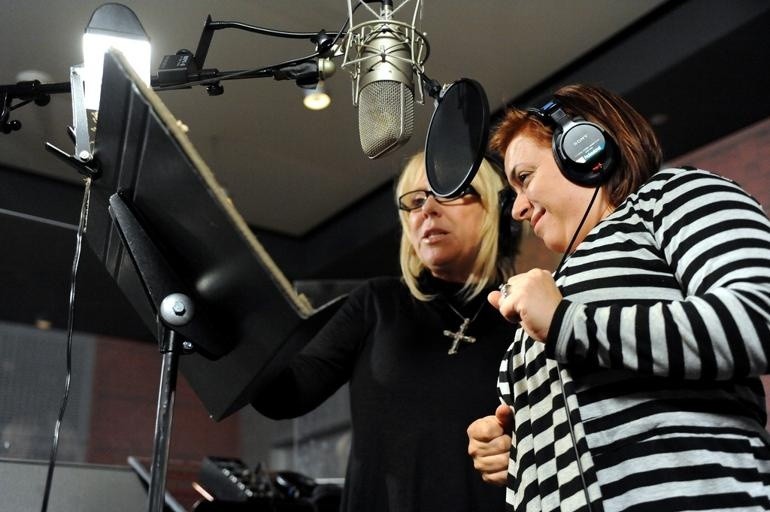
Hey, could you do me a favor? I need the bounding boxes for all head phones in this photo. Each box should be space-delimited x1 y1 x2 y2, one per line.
466 145 522 244
528 103 616 187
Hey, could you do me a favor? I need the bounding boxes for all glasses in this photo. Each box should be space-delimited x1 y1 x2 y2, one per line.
399 184 478 210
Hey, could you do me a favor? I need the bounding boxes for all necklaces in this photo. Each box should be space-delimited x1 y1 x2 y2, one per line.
438 290 489 358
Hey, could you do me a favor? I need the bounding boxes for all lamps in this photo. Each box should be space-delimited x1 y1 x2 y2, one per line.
303 80 331 112
69 3 152 163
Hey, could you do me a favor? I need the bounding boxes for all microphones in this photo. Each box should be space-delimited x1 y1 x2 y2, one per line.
356 0 416 162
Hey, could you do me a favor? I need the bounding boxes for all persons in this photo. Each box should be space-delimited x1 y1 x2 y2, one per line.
251 149 512 511
465 83 770 512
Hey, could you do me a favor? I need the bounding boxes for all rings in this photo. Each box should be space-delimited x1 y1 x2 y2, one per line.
498 281 511 299
